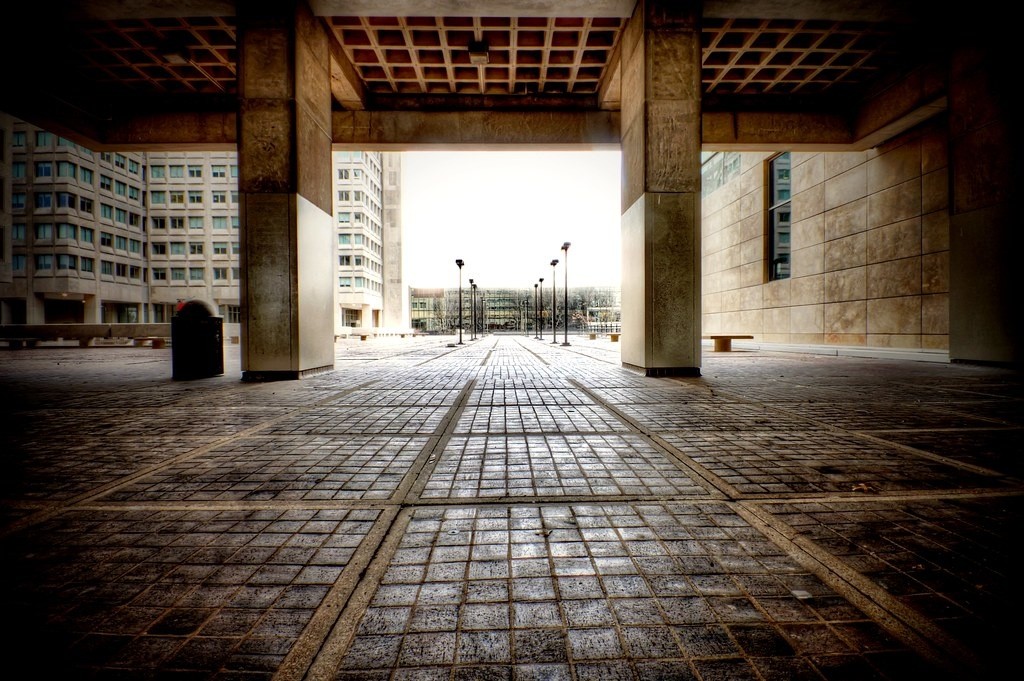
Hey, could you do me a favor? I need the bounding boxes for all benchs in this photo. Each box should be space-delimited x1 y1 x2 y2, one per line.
588 332 602 340
607 334 620 342
134 337 171 350
702 335 754 351
334 333 430 342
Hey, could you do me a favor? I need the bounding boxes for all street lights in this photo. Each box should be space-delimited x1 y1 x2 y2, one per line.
472 284 477 339
538 277 544 340
551 259 560 344
468 278 474 341
534 283 539 339
455 258 464 345
561 242 571 345
